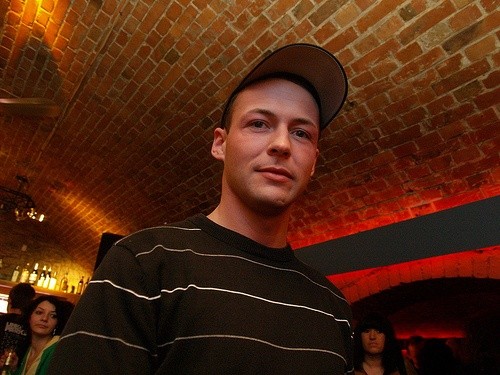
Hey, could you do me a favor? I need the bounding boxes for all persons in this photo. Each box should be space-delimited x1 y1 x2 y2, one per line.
354 316 458 375
0 283 74 375
47 43 356 375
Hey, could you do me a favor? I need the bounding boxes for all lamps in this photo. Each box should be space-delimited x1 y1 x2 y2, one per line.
0 176 45 223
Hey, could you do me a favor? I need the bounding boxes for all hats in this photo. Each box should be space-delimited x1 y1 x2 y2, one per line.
220 42 350 132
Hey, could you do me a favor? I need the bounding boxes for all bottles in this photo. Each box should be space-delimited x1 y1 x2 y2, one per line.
37 265 76 296
28 262 39 285
84 278 90 291
76 276 84 295
0 346 16 375
19 262 30 284
10 265 20 283
